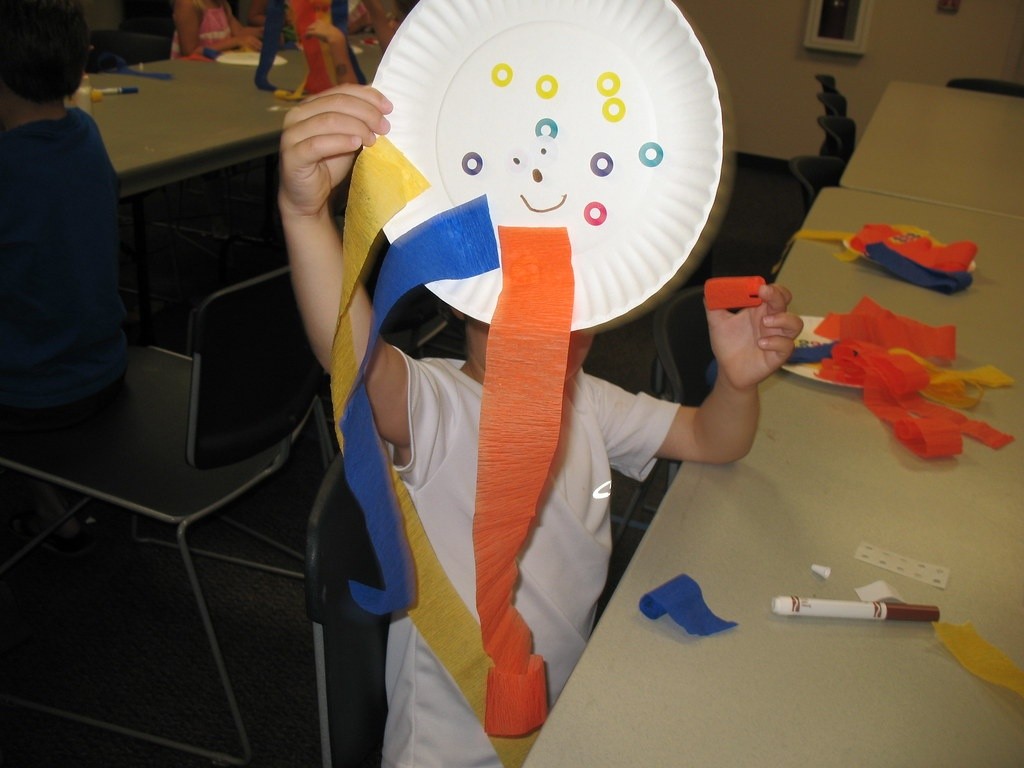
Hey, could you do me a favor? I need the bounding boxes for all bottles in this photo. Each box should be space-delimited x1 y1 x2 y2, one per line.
74 77 93 115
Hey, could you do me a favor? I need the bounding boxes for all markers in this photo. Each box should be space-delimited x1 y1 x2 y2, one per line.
94 85 138 97
773 597 941 624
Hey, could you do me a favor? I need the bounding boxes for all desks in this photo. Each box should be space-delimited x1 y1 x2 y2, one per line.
61 75 299 198
117 29 382 100
520 186 1024 767
838 80 1024 219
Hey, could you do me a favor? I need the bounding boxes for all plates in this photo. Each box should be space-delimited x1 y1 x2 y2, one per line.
843 239 976 271
782 316 862 388
364 0 724 333
217 52 287 66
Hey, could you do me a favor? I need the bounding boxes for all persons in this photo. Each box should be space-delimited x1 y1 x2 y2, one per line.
169 0 284 239
276 84 805 768
306 0 419 86
1 0 130 557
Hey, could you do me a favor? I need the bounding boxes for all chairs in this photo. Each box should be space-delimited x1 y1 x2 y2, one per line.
304 454 388 768
611 73 858 547
0 262 334 764
